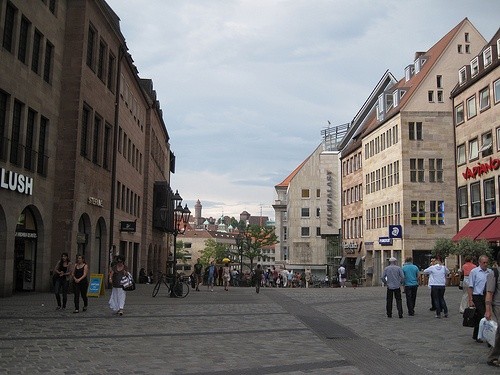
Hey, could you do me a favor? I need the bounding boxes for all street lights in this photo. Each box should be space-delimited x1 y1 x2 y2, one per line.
160 189 191 298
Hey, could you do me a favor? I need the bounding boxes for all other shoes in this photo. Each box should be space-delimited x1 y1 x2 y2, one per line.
83 304 88 311
399 315 403 318
473 337 484 343
61 307 65 312
56 305 61 310
488 360 500 367
429 307 437 311
120 311 123 315
387 314 392 318
75 310 79 313
435 314 441 318
444 313 447 317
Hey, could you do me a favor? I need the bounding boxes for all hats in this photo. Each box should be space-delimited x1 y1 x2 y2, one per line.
389 257 397 262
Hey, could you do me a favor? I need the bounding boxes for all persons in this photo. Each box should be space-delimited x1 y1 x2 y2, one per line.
222 263 230 292
337 265 347 288
484 253 499 367
139 268 150 285
468 255 493 343
253 265 264 294
54 253 72 311
422 255 449 318
401 257 420 316
380 257 405 318
72 255 89 313
460 254 477 313
261 267 313 288
205 260 217 292
192 258 204 292
108 255 129 316
232 269 240 287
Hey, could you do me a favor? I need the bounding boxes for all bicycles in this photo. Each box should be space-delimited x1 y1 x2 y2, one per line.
151 271 190 300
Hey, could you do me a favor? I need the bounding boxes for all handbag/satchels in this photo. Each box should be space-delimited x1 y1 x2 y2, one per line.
477 317 498 348
463 305 479 327
120 271 136 291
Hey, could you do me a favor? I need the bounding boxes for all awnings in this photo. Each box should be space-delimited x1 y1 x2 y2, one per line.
451 217 496 245
475 216 500 242
